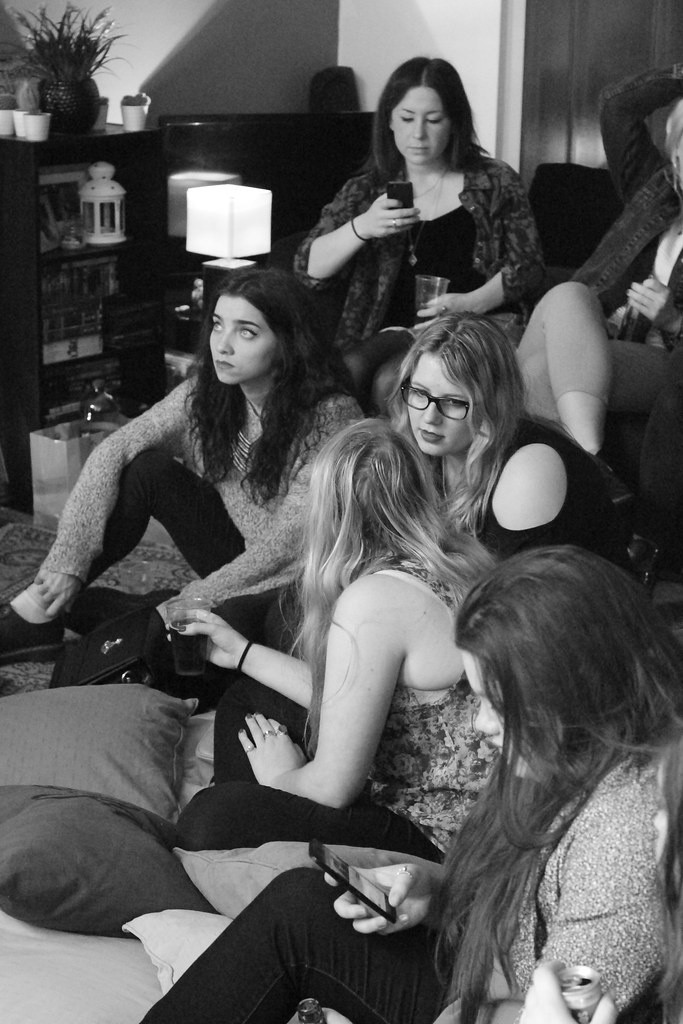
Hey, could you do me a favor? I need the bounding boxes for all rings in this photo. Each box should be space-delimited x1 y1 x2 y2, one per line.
245 745 254 753
393 219 396 227
276 725 288 736
397 866 413 878
263 731 277 740
441 306 447 313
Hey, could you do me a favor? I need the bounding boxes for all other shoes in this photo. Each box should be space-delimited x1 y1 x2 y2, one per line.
0 601 65 665
588 447 635 506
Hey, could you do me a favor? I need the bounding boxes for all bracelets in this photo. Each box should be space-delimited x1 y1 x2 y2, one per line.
351 217 368 241
236 641 256 672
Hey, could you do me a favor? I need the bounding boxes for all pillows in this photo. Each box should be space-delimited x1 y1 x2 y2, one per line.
2 686 439 1023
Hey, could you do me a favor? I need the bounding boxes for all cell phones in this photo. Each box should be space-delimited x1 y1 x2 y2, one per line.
387 181 415 211
308 837 398 926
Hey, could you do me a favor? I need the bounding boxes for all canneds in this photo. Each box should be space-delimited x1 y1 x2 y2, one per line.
554 965 602 1024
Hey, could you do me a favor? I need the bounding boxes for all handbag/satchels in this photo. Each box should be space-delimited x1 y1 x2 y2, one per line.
49 601 166 688
28 420 119 535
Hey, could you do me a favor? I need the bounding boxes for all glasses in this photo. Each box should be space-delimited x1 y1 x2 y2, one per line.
400 375 477 420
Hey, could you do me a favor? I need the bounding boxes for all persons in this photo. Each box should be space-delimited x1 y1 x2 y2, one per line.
133 546 683 1024
0 56 683 667
165 418 503 863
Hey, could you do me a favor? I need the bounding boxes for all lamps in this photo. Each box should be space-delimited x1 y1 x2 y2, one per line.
186 184 274 340
78 161 128 244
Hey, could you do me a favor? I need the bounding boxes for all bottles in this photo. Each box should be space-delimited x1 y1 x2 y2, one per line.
297 998 327 1024
81 379 123 438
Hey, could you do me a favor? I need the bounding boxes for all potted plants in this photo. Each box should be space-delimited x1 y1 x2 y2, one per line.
120 93 149 133
1 77 53 140
92 97 109 131
10 5 131 134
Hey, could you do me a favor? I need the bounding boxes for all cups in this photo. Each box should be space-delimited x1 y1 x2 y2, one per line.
165 597 212 675
412 274 452 323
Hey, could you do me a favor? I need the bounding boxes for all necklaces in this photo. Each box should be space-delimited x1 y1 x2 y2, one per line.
441 457 448 503
246 399 260 418
407 168 448 265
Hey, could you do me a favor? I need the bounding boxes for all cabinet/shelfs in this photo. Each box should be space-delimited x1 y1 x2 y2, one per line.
2 109 374 511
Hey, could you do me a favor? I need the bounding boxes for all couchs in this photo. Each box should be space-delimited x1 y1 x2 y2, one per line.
530 160 682 523
